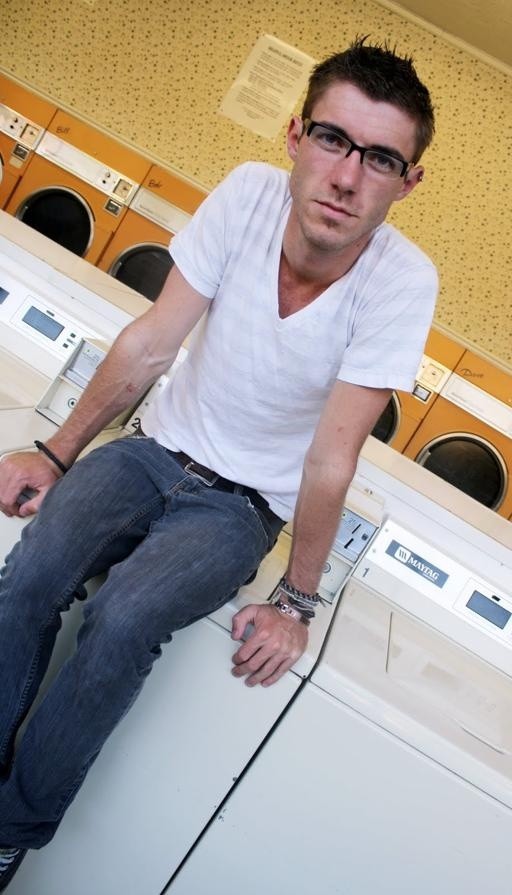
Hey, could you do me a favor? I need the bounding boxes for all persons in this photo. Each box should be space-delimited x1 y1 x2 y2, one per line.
0 34 439 895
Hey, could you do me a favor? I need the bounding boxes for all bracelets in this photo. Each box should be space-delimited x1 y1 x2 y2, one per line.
33 439 69 475
267 577 331 627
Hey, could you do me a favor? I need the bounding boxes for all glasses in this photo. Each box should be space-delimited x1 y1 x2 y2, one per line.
300 115 408 185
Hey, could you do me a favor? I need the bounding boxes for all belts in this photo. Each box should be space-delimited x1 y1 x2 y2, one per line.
134 424 287 531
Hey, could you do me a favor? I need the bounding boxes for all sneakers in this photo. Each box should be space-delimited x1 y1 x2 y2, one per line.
0 844 27 892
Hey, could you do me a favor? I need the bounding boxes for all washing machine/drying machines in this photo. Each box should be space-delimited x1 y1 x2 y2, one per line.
2 69 511 519
3 207 511 894
